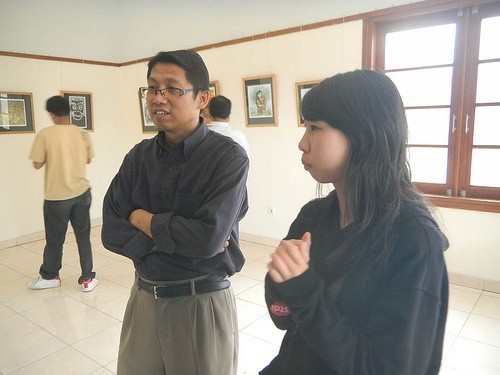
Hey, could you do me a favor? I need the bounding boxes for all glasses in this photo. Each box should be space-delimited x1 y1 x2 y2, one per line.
138 87 198 101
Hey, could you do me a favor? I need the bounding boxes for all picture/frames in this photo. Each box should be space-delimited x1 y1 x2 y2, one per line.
198 80 221 128
295 80 322 128
61 91 94 133
242 74 278 128
0 91 36 134
140 87 161 134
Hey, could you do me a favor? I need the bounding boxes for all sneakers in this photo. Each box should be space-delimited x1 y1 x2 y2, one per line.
81 278 99 291
28 275 62 289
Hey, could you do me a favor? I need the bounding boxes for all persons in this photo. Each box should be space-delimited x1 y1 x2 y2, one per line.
259 69 450 375
101 49 250 375
204 96 249 159
27 96 99 292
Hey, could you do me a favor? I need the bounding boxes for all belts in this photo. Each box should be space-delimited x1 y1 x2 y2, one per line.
135 271 230 300
